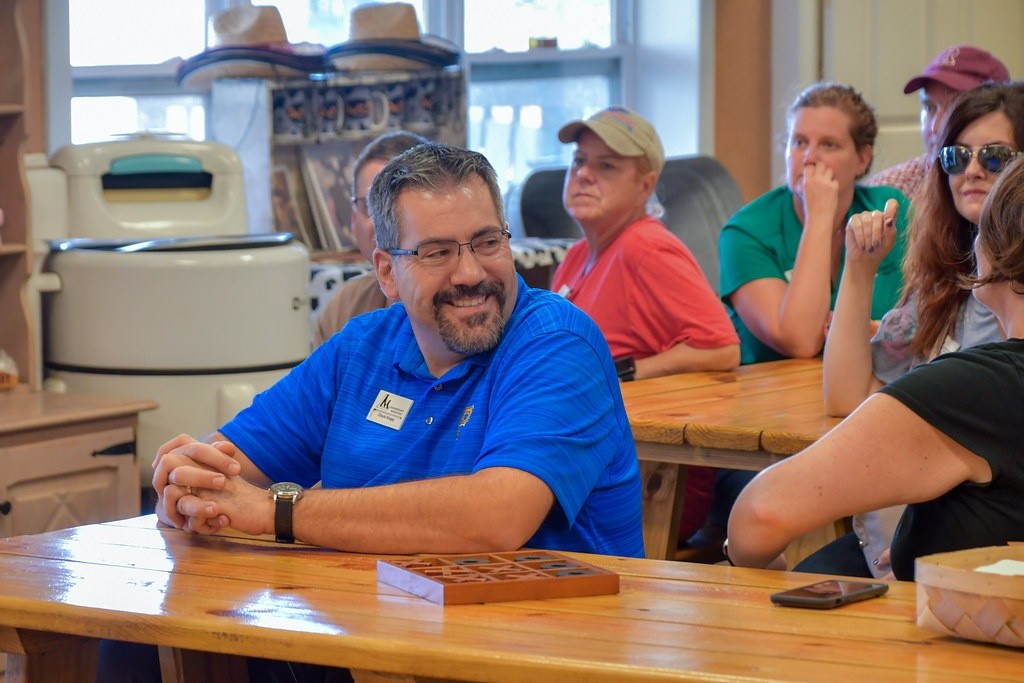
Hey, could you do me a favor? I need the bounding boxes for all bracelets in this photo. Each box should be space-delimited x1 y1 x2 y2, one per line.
723 538 735 567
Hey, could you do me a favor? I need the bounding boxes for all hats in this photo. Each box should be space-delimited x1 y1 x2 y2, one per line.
175 7 323 93
904 45 1011 94
558 106 665 184
326 2 460 72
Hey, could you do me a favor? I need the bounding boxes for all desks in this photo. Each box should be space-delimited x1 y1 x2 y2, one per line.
0 513 1024 683
617 351 846 572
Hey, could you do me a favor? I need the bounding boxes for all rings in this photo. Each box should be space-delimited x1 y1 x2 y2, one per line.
186 486 192 494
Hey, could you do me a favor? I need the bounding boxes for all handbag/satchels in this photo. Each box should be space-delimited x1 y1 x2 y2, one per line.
852 504 909 579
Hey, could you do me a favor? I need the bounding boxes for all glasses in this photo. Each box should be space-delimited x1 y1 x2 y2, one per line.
353 196 370 218
939 144 1022 176
385 228 511 267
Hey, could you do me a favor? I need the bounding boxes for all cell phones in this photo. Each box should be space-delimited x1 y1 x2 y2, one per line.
770 579 889 610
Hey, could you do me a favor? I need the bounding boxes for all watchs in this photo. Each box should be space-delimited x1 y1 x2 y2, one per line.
615 356 636 382
268 482 304 544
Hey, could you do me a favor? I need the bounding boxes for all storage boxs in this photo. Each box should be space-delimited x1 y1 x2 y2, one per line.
376 549 620 606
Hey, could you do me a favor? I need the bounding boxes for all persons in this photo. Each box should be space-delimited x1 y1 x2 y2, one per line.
311 132 429 354
552 104 739 539
98 143 646 683
791 81 1024 579
723 157 1024 582
718 84 916 515
864 47 1012 198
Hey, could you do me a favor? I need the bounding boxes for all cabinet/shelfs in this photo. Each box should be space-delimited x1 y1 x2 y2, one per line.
1 0 160 539
210 49 582 350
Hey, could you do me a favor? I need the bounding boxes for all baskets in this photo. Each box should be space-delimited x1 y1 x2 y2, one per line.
914 542 1024 647
